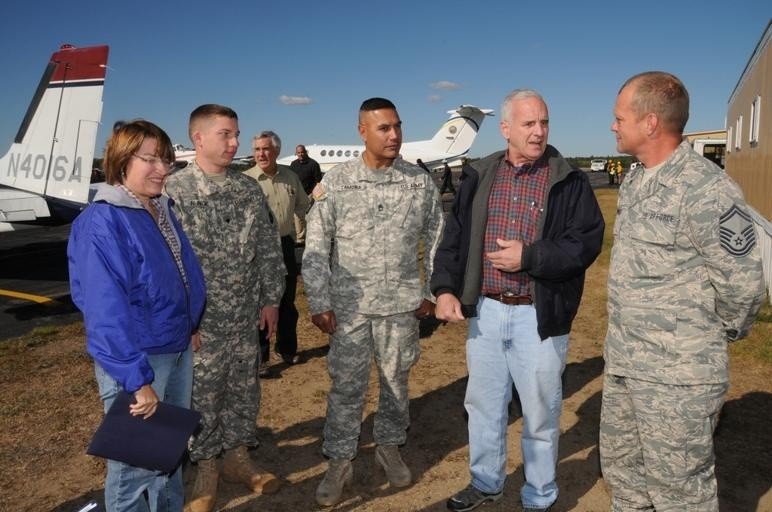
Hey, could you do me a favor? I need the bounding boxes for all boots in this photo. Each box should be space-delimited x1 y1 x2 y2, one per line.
189 457 218 512
315 459 352 507
222 447 278 493
374 444 410 487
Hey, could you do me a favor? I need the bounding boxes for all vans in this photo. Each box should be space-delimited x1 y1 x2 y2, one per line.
590 159 607 172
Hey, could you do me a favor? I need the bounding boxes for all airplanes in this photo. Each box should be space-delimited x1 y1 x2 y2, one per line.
275 103 496 172
0 43 110 232
172 142 254 164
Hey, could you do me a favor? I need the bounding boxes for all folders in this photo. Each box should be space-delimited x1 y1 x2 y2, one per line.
85 391 203 475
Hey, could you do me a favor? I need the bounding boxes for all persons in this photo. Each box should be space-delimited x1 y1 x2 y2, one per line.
241 130 312 377
597 71 765 511
67 122 208 511
303 96 446 508
416 157 622 197
290 145 323 244
161 104 288 510
430 89 605 510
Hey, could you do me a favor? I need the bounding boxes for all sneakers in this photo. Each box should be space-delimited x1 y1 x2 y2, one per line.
258 361 269 378
282 352 301 364
447 484 504 511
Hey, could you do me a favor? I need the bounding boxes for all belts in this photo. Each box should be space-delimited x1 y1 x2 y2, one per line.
486 293 533 305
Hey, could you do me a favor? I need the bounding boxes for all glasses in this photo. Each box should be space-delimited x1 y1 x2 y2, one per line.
134 154 170 165
